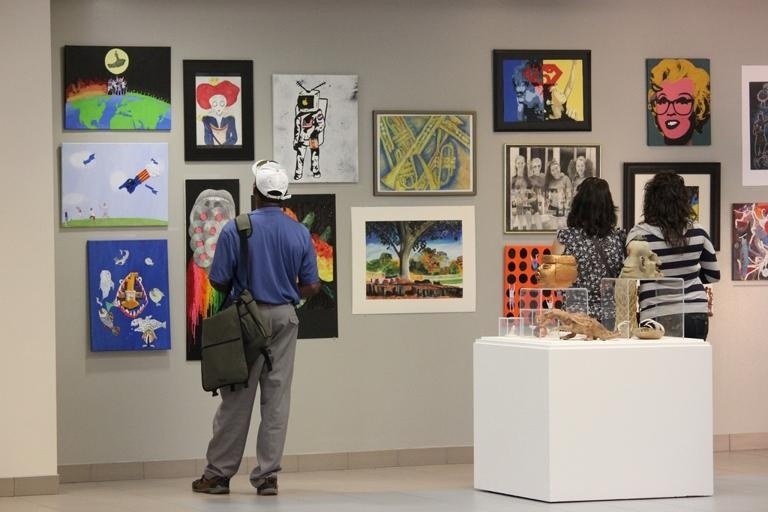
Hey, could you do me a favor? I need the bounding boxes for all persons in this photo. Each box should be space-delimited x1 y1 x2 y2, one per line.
190 159 322 499
626 170 720 336
535 255 577 289
105 75 127 96
510 61 566 125
198 80 239 146
552 176 628 320
511 155 589 230
647 59 710 145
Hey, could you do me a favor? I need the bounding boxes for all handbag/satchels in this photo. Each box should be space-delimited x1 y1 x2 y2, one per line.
198 213 275 398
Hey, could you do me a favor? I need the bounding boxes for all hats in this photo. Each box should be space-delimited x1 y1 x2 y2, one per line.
251 158 292 201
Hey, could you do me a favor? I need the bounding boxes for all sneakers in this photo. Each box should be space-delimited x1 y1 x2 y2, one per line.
257 478 279 497
191 475 231 495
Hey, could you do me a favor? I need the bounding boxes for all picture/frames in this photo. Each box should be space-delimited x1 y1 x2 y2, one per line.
739 63 768 188
623 163 721 250
371 108 477 198
492 47 592 132
348 204 479 314
183 58 255 161
503 143 603 235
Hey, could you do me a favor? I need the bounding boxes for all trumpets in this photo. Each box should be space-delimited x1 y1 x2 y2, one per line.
376 116 471 190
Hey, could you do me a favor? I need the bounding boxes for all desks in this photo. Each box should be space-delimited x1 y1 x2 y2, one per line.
472 330 714 504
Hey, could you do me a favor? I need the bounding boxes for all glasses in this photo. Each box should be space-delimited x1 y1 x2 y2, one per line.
650 91 697 117
254 159 281 171
